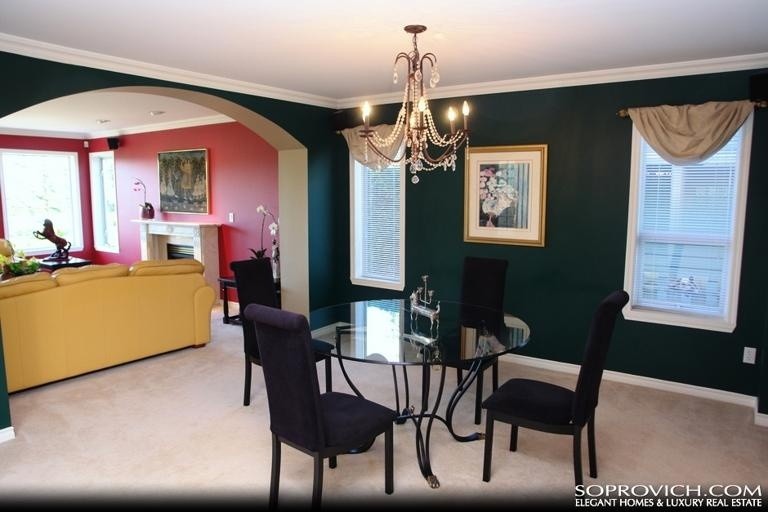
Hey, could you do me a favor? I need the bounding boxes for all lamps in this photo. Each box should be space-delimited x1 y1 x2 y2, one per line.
359 25 470 183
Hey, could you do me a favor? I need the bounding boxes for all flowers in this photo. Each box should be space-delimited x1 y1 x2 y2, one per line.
256 205 279 263
128 177 153 211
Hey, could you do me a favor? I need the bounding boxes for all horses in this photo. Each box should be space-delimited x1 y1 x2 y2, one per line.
33 219 71 259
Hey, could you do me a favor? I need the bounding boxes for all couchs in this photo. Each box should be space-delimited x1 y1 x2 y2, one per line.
0 258 217 394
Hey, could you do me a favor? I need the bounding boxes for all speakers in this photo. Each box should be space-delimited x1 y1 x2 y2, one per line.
107 139 118 149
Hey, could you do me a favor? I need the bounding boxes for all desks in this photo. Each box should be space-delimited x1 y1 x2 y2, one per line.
218 276 280 325
304 298 531 489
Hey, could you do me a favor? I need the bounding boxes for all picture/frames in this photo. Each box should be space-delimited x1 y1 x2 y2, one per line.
157 148 210 215
463 144 548 248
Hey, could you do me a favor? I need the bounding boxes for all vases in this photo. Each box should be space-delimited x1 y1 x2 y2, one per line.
141 209 154 219
